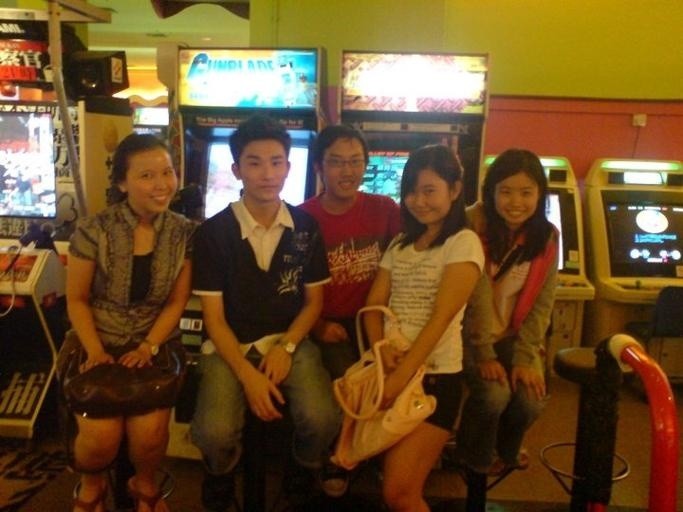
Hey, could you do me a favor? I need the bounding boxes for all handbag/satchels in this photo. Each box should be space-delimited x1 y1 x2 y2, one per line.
63 338 182 419
328 303 437 471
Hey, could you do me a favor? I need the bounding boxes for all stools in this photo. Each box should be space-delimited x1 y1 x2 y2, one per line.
540 343 639 495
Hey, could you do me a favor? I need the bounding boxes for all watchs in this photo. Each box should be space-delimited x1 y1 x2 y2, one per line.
271 336 298 355
142 337 160 359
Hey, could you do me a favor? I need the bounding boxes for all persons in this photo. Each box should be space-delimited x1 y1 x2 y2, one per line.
192 108 343 507
61 134 196 511
315 144 482 511
458 144 563 474
288 120 411 381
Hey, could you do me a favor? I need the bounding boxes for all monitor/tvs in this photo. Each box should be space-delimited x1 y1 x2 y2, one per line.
543 192 563 271
0 110 56 219
202 126 317 222
357 146 410 205
600 190 683 278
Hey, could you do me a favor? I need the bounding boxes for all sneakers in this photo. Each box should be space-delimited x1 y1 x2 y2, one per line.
509 443 528 470
475 455 502 477
201 475 234 510
317 464 346 499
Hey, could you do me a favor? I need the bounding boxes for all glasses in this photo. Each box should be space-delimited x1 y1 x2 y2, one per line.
320 157 366 169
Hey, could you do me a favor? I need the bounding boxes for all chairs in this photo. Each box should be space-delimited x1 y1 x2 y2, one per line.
625 285 683 404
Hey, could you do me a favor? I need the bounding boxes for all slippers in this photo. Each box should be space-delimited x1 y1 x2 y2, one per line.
127 474 169 512
72 479 107 512
